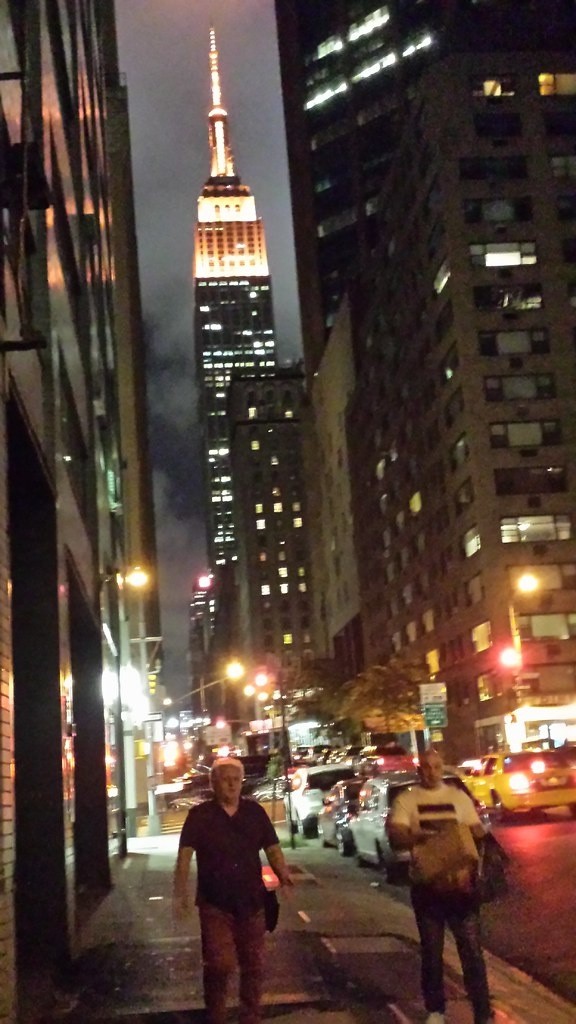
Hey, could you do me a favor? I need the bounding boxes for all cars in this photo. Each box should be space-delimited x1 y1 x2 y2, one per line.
288 743 417 776
315 776 377 857
345 770 491 886
280 763 356 840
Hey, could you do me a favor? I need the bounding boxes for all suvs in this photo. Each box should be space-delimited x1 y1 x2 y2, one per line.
460 750 576 823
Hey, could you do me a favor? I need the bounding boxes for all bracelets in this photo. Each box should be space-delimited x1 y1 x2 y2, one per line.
280 879 295 889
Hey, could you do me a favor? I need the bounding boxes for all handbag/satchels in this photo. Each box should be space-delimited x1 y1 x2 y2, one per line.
471 831 510 901
258 886 280 934
411 821 481 880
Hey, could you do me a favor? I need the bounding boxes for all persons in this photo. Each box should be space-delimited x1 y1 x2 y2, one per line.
387 749 495 1023
171 757 299 1024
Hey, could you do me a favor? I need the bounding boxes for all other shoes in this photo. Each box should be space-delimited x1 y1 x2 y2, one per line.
424 1011 445 1024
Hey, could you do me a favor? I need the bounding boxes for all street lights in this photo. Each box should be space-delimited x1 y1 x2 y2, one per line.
122 561 161 836
509 573 542 706
